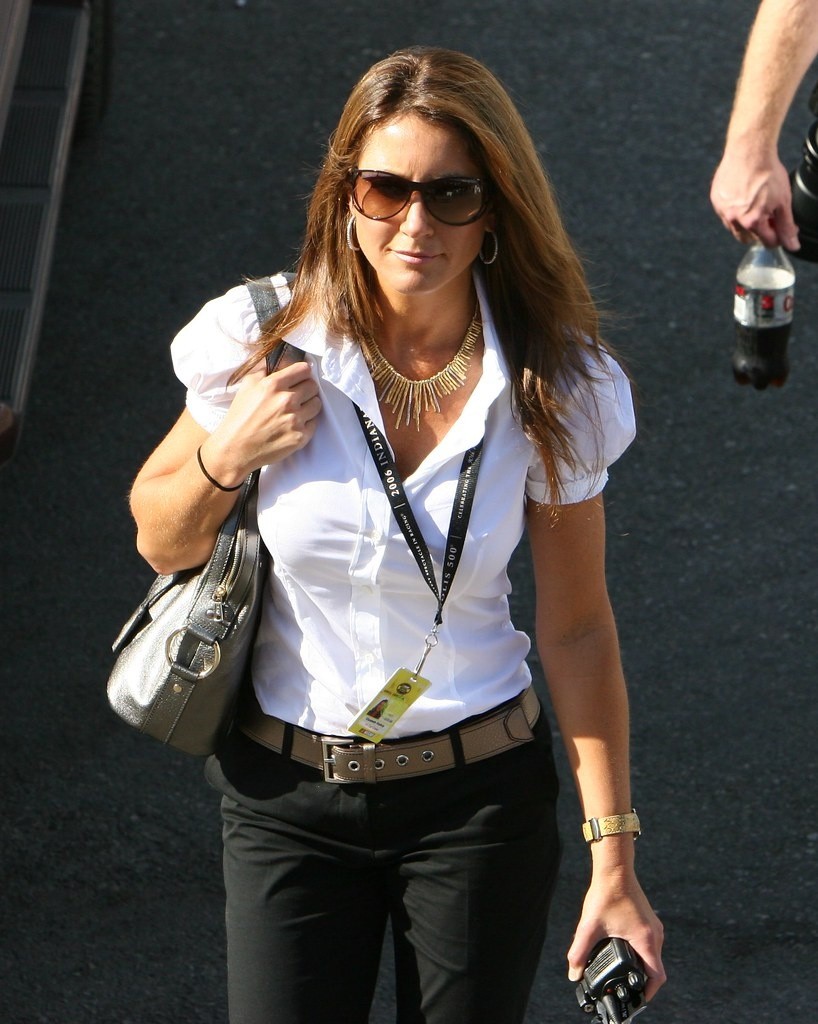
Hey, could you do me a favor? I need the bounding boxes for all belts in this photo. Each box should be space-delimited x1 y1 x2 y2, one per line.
242 682 541 784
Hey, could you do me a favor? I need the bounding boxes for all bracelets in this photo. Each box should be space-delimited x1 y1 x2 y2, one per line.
196 446 245 492
582 808 642 843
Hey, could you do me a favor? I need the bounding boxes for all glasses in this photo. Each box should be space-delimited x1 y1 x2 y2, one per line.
345 166 491 227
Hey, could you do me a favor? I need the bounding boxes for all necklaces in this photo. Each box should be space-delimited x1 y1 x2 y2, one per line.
354 303 482 431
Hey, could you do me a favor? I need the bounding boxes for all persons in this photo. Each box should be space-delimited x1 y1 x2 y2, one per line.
711 0 818 264
368 699 389 719
129 48 667 1024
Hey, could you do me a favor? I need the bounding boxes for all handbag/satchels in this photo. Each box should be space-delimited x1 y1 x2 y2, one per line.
106 275 284 753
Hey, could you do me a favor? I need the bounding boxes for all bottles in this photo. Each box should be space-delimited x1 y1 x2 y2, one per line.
731 218 798 392
